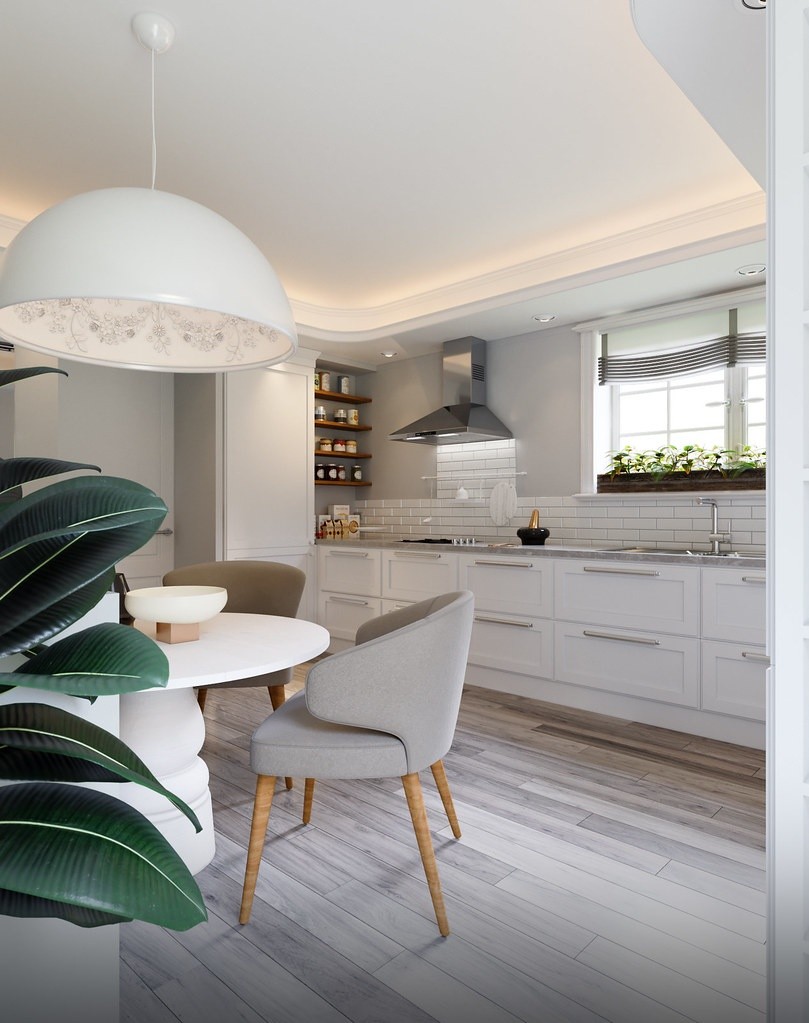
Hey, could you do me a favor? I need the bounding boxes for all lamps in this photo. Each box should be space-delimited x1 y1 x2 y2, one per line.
0 12 300 372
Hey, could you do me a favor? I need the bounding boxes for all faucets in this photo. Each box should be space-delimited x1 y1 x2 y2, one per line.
696 497 732 556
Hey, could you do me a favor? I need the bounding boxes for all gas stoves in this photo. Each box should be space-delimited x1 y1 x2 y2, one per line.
394 538 480 545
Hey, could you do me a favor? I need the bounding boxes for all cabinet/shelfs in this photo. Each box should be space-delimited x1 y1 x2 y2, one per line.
314 389 372 487
317 547 765 722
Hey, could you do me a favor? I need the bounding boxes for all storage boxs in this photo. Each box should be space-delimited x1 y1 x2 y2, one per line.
317 504 360 540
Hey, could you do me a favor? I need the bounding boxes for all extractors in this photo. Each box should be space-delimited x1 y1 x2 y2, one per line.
387 337 514 446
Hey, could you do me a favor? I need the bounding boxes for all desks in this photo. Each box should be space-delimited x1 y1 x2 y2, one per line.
119 612 329 873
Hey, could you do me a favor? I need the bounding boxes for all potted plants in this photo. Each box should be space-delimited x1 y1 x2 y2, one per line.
597 444 766 493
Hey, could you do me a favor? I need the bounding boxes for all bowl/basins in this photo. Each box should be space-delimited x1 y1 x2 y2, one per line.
126 585 228 624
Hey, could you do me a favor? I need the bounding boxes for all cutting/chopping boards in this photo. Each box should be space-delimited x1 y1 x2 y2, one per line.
489 482 518 526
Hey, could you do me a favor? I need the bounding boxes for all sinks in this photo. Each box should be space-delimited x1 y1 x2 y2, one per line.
596 547 693 556
735 551 766 560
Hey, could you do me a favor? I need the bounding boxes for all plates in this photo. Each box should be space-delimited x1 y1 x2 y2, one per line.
352 527 387 531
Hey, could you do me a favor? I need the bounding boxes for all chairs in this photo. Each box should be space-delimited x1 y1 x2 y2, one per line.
239 591 473 937
163 558 305 712
